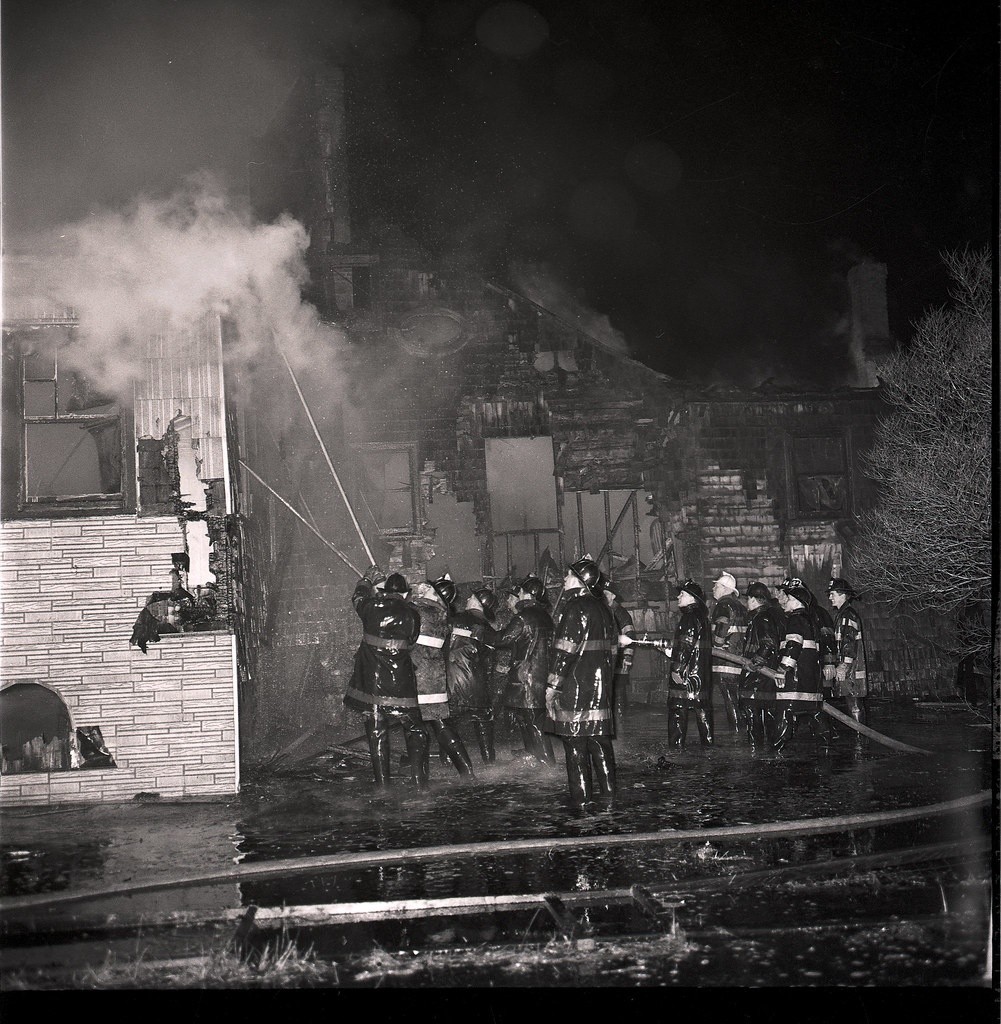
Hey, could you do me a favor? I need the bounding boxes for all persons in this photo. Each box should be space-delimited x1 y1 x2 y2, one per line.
345 550 871 804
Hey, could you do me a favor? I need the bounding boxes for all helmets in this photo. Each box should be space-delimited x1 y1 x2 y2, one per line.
782 578 810 593
513 577 546 602
536 587 552 608
746 581 772 601
385 572 412 593
712 571 739 597
784 588 812 613
676 583 706 604
824 578 857 594
600 578 624 603
569 559 605 599
427 579 456 610
776 577 791 590
469 586 499 622
505 580 524 598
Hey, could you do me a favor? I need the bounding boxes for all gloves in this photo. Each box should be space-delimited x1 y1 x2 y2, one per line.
822 665 838 681
544 686 563 722
740 654 767 684
672 671 685 687
774 666 786 688
622 655 633 672
469 624 485 640
363 565 386 584
834 663 849 682
